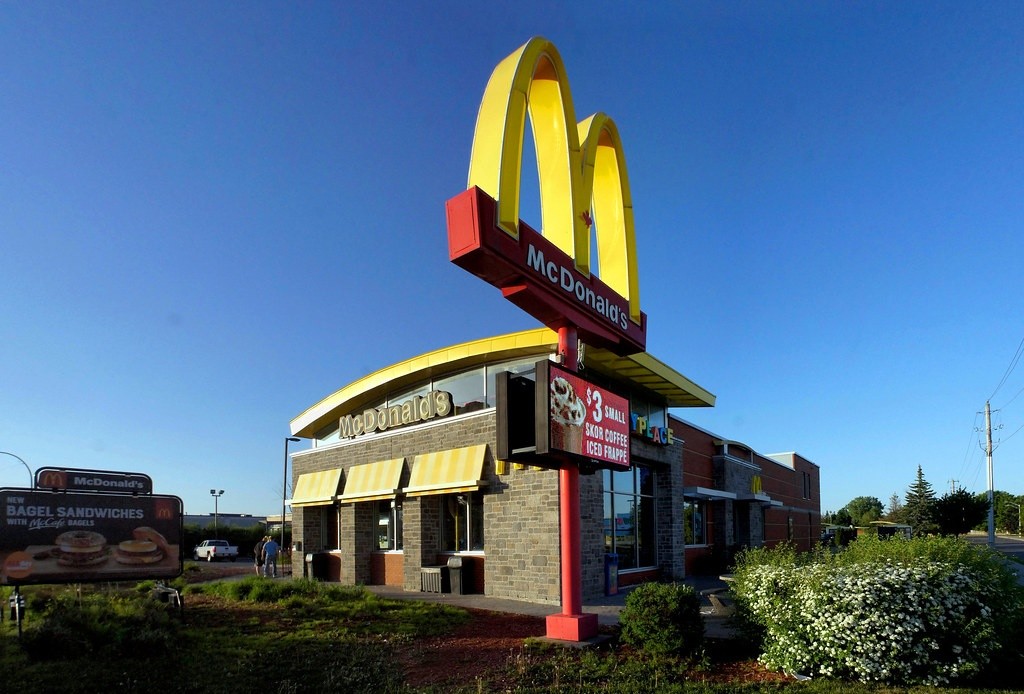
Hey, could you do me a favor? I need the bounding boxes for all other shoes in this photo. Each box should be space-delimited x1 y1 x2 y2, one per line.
273 573 279 578
264 574 269 578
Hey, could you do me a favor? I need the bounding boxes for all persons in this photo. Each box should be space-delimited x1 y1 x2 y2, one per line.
253 535 278 578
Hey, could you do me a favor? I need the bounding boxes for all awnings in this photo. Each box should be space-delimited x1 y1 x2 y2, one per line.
291 444 489 509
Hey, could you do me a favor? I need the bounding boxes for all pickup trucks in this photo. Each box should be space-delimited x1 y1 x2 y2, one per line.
192 540 239 563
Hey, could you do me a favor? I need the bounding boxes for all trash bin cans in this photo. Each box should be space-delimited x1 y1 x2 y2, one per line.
420 565 450 594
306 553 328 582
447 556 474 594
604 554 618 597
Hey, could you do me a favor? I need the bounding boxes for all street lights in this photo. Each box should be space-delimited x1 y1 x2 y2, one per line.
278 437 302 561
210 489 225 539
